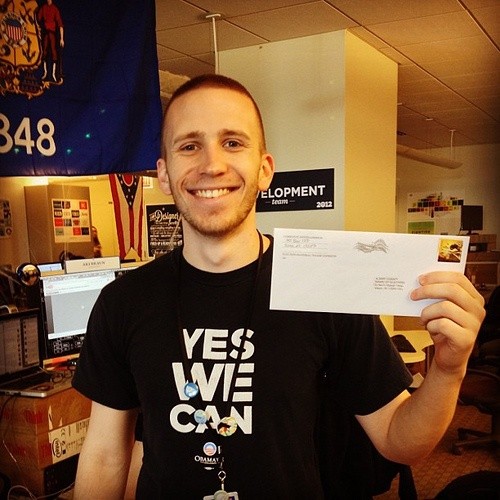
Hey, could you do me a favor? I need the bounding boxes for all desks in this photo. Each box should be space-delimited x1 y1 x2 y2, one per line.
388 330 434 373
0 366 91 500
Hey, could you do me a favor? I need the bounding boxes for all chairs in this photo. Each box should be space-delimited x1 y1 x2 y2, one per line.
453 285 500 458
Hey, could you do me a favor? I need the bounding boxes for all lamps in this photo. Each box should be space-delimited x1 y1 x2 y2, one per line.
396 128 462 169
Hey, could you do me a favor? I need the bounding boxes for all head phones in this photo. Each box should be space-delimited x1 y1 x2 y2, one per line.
16 261 41 286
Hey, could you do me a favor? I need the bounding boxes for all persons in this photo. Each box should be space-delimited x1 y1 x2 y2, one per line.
91 225 102 258
70 73 486 500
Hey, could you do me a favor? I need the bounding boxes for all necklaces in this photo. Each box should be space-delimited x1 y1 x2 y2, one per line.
176 228 263 500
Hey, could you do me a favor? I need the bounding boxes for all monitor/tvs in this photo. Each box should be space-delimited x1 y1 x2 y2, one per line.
460 205 484 234
31 258 120 373
